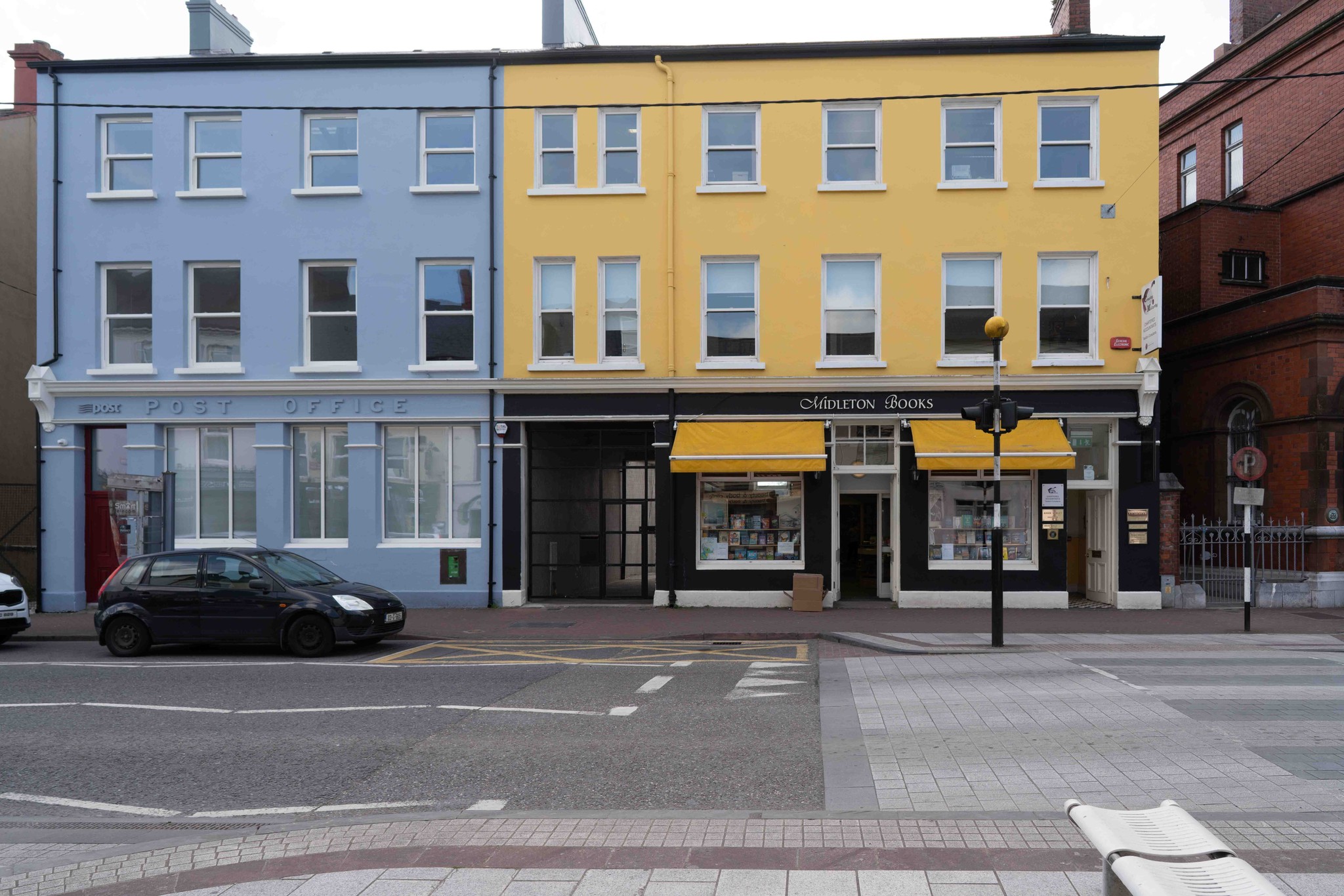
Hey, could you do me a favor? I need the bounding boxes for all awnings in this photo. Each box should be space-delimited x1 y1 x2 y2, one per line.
910 419 1077 470
669 421 828 473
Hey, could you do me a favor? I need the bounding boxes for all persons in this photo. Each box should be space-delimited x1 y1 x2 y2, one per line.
731 533 737 543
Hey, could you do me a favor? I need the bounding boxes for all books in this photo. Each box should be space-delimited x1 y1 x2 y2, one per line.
942 514 1026 560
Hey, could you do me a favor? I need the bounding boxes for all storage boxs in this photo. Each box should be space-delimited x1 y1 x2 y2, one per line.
762 518 770 528
783 573 829 611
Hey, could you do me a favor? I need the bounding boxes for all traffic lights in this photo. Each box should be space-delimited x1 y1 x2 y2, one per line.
1000 401 1034 429
960 401 992 430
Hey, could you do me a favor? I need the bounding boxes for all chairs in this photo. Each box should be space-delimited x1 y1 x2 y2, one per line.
208 559 234 588
238 560 258 582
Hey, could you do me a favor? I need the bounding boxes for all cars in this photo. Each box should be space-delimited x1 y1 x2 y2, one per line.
0 569 31 644
94 546 407 658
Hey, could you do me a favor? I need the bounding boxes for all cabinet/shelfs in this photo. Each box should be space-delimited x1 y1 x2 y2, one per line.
929 528 1030 560
701 527 801 560
